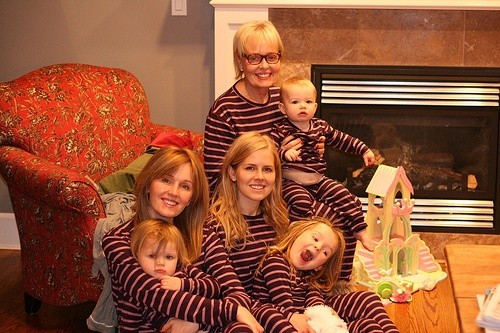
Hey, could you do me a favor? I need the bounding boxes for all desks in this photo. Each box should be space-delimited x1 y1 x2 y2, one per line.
442 242 500 333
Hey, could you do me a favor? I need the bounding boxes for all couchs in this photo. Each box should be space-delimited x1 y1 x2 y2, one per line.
0 63 205 316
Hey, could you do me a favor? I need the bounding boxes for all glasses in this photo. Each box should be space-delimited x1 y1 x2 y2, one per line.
242 50 281 65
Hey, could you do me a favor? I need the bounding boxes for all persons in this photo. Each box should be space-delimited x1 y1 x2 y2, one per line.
101 19 400 333
